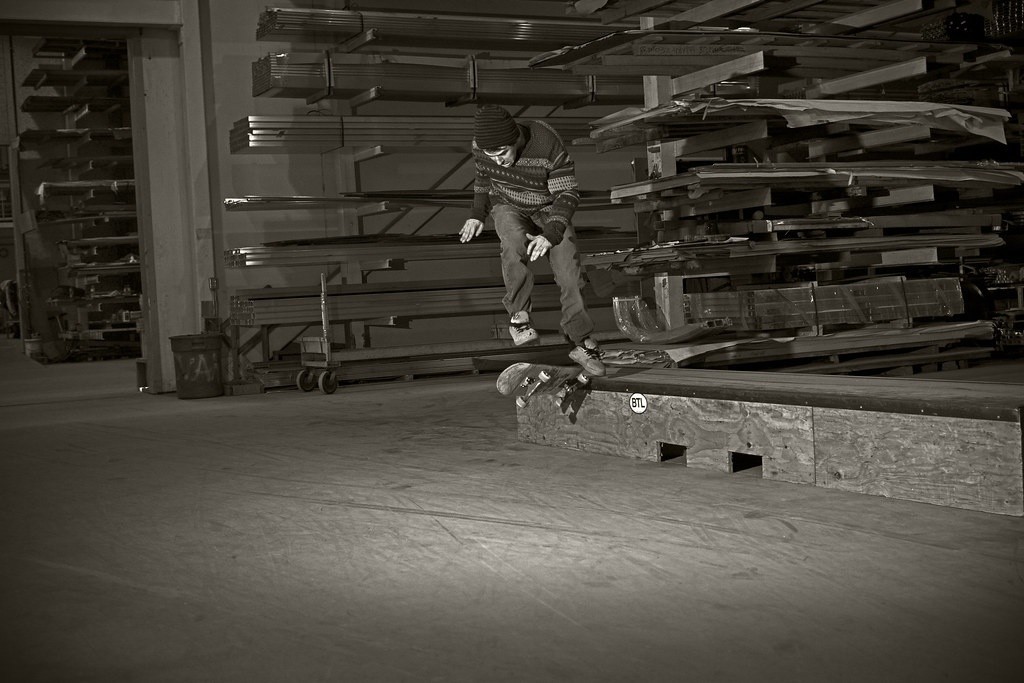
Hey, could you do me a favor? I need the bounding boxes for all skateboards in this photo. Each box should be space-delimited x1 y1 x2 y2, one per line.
496 361 590 409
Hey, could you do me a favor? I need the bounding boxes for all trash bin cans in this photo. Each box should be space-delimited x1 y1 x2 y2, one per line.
169 334 222 400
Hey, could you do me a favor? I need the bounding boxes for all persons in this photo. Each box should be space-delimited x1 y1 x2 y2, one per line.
458 104 607 376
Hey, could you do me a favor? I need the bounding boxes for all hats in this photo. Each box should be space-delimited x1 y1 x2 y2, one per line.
474 104 520 149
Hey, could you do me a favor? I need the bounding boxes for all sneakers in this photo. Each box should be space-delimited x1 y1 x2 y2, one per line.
508 311 539 347
568 336 608 378
